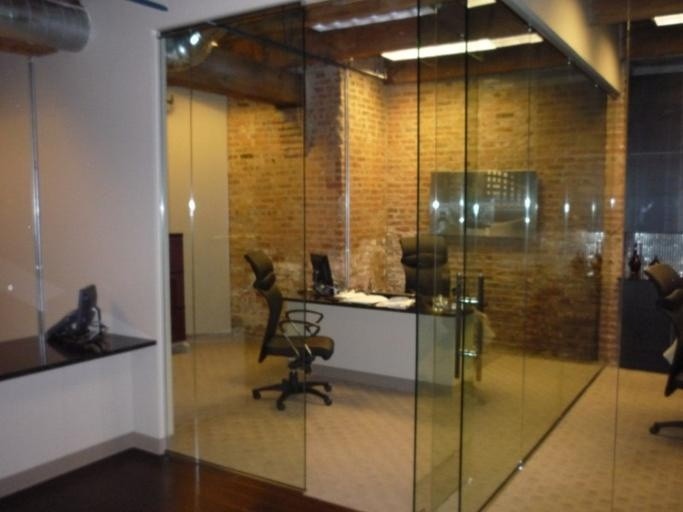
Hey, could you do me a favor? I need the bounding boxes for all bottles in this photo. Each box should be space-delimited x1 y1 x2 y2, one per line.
628 242 640 274
648 255 659 281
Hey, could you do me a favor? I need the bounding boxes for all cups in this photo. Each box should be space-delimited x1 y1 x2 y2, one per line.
432 295 448 315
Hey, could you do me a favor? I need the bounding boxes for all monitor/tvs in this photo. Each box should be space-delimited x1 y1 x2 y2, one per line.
310 254 334 296
74 285 104 346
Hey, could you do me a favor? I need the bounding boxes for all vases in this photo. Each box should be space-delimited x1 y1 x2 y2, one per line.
629 249 641 277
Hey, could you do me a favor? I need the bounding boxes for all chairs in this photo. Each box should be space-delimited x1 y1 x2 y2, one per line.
399 235 452 300
243 248 337 412
642 263 683 438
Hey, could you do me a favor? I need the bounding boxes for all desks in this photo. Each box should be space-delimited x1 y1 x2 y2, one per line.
279 288 488 401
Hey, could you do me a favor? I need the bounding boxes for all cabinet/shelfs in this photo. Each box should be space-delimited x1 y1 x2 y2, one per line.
619 276 675 375
